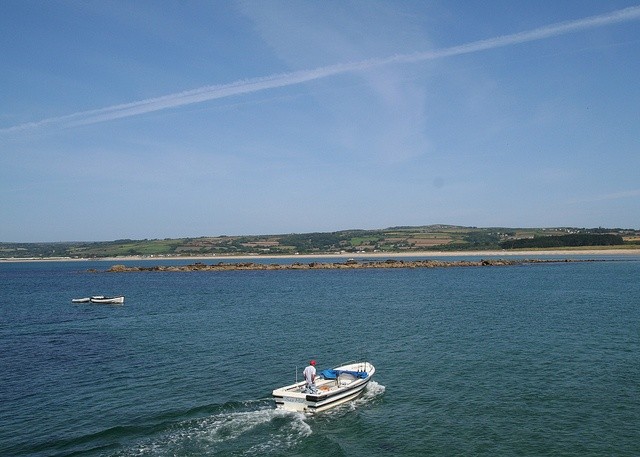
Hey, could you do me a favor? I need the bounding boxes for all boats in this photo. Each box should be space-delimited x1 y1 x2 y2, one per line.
272 361 376 417
71 295 106 303
90 296 126 304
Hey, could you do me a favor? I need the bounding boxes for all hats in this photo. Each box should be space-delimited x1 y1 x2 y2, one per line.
310 360 316 364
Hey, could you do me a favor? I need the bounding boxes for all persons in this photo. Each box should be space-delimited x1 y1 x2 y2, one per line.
303 360 316 388
